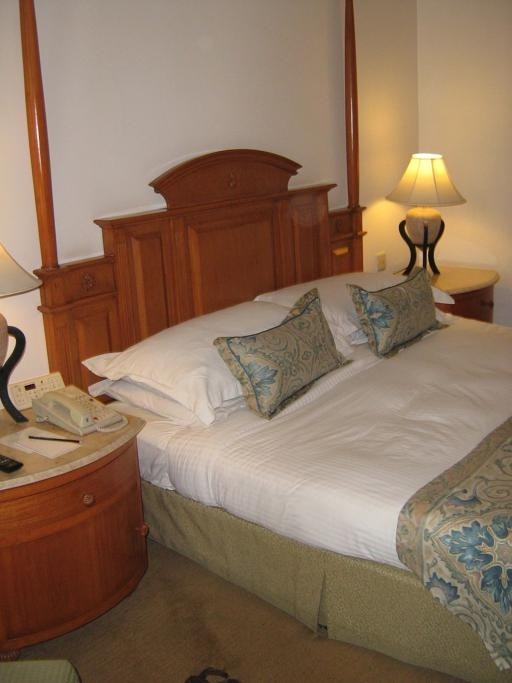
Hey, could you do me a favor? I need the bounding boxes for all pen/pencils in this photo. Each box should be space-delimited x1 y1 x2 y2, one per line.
29 436 80 444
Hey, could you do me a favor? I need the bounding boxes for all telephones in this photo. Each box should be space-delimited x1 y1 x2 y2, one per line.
32 385 128 435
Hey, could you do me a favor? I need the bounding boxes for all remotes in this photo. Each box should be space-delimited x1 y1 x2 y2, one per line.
0 454 24 473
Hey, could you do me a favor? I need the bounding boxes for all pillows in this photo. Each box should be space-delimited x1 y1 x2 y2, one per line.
254 264 455 333
347 305 458 344
79 301 349 414
346 266 449 360
212 287 352 421
86 378 250 429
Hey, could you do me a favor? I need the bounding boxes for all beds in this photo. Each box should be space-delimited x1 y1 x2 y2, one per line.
35 148 512 682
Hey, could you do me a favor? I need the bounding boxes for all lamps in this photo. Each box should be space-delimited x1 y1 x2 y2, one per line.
0 240 43 425
385 151 467 276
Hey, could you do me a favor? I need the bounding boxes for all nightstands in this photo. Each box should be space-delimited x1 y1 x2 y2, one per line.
0 401 148 649
393 266 500 322
